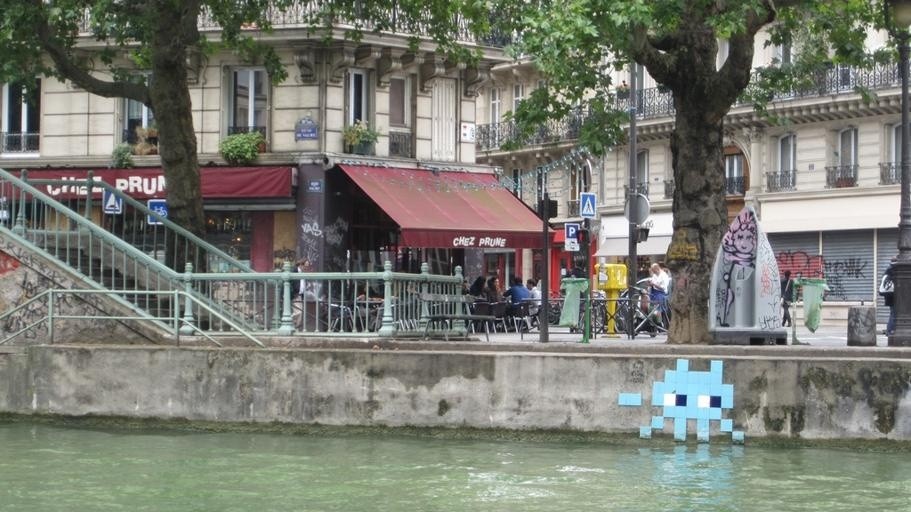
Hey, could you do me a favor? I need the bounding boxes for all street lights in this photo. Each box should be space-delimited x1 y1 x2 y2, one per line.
882 1 910 344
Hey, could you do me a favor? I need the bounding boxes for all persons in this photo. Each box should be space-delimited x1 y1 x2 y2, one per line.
780 270 799 327
646 263 669 312
879 256 898 337
462 275 542 332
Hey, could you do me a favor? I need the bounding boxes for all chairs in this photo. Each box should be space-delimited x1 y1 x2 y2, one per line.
375 301 408 333
323 301 354 332
466 302 540 334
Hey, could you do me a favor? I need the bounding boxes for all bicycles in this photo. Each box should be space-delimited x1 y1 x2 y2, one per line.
546 287 662 337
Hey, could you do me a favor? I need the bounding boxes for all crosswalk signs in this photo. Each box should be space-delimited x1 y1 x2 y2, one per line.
579 193 596 221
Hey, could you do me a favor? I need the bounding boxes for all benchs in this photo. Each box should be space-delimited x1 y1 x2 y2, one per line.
415 292 496 341
